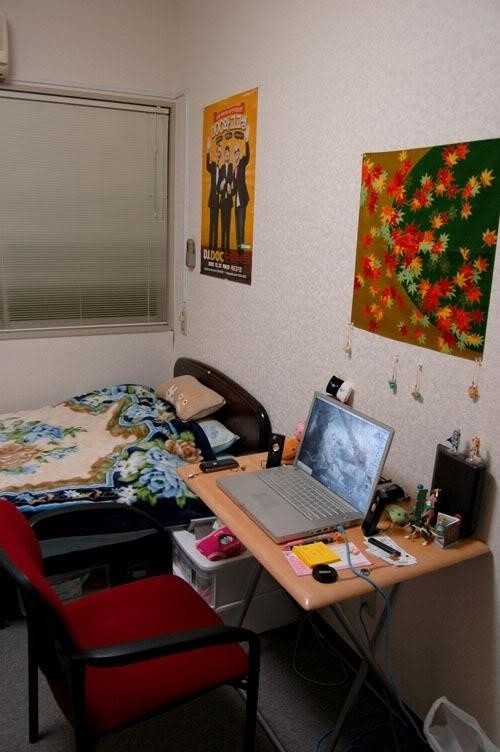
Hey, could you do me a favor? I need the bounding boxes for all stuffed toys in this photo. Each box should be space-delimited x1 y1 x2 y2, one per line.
281 420 306 465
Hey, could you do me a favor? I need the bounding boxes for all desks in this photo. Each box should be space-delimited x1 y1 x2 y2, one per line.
175 452 488 752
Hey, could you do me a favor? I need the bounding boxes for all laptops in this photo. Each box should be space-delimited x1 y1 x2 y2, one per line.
217 391 395 545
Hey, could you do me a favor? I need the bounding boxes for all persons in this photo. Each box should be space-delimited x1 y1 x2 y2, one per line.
206 125 250 256
451 428 461 453
404 484 440 546
469 436 481 462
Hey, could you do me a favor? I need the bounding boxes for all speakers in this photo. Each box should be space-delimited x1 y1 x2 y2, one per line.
266 433 285 468
361 489 389 537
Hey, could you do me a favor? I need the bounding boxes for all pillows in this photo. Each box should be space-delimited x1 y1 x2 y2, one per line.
197 418 240 455
155 375 225 423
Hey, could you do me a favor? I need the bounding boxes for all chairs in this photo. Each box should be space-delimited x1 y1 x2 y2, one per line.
0 497 260 752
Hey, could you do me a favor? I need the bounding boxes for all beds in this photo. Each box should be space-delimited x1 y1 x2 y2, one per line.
0 357 271 563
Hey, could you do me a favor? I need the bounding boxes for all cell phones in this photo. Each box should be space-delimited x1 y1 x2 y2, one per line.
200 458 239 473
